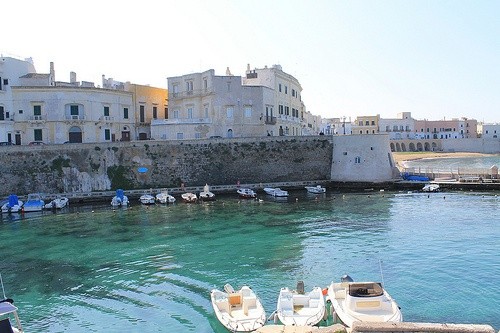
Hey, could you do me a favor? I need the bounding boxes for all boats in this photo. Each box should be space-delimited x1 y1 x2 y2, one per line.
236 187 257 199
210 282 267 333
421 182 441 192
198 183 216 202
110 188 130 207
155 191 177 204
21 193 45 212
263 187 289 197
304 184 327 193
0 192 24 213
138 194 156 205
181 192 198 203
44 196 69 210
276 281 326 327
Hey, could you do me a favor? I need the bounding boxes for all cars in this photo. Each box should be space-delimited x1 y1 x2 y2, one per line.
0 141 15 146
28 141 47 145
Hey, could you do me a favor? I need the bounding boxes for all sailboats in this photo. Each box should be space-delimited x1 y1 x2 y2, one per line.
328 259 403 327
